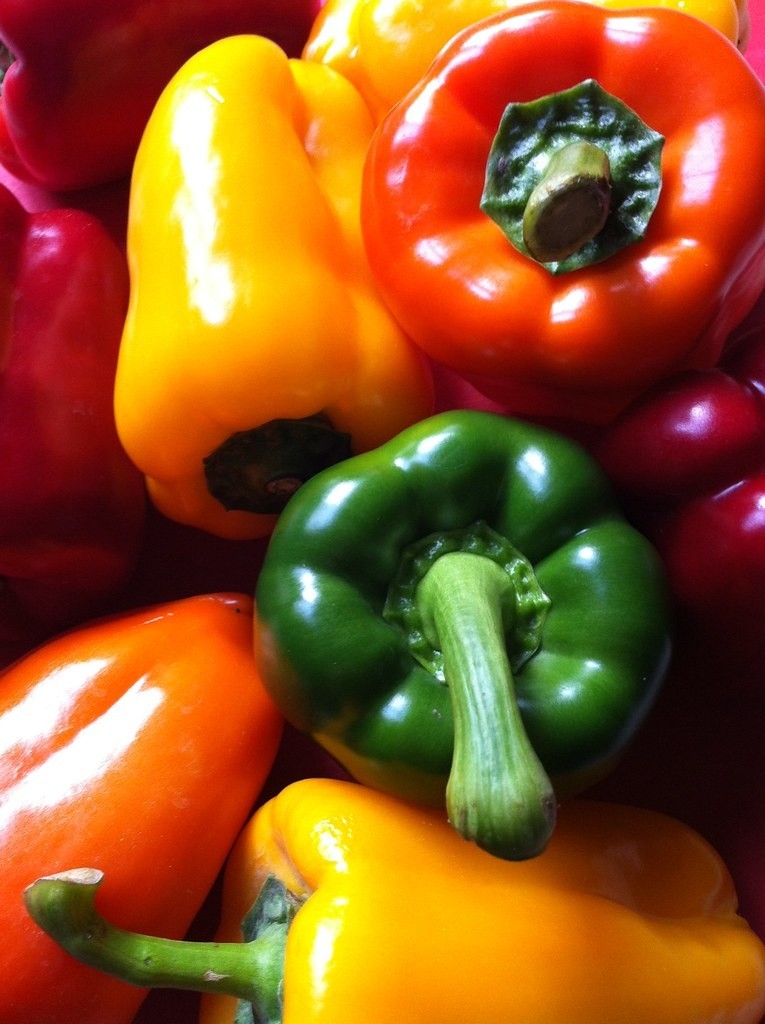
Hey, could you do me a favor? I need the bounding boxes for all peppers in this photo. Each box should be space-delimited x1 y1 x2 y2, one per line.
0 1 765 1024
